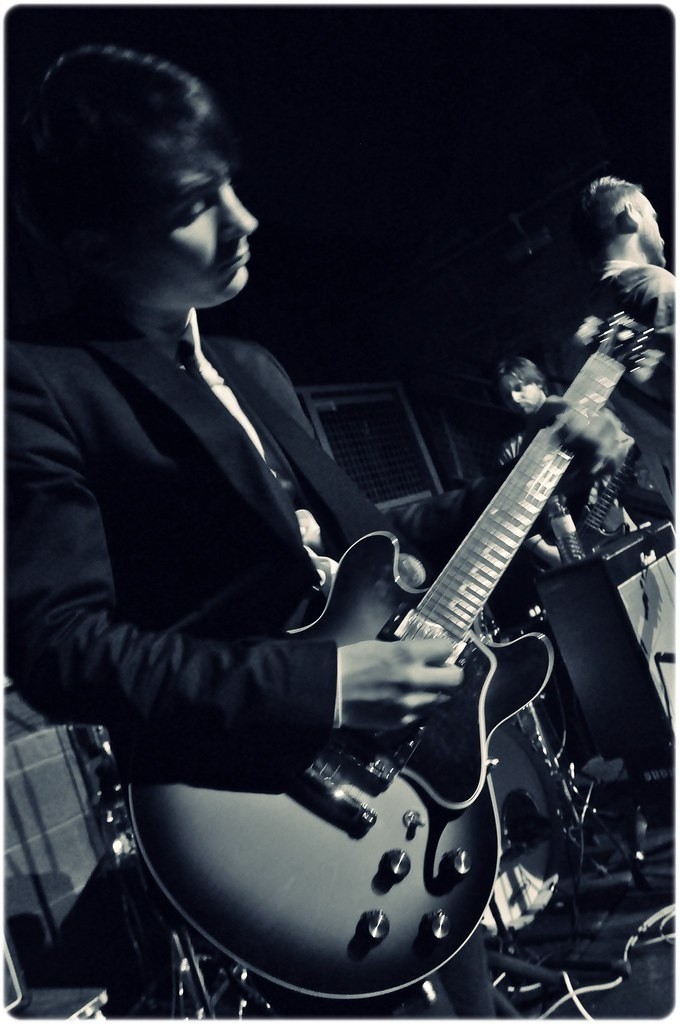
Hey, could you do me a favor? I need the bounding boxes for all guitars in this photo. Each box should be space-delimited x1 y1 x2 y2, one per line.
574 442 643 556
122 309 666 1001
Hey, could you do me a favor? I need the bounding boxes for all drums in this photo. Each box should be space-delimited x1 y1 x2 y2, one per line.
465 720 566 935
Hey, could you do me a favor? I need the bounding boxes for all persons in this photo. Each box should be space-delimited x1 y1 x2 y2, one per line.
5 48 622 1020
583 175 675 429
496 356 626 567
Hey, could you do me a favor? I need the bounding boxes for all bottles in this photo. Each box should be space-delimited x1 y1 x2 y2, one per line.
548 495 586 565
